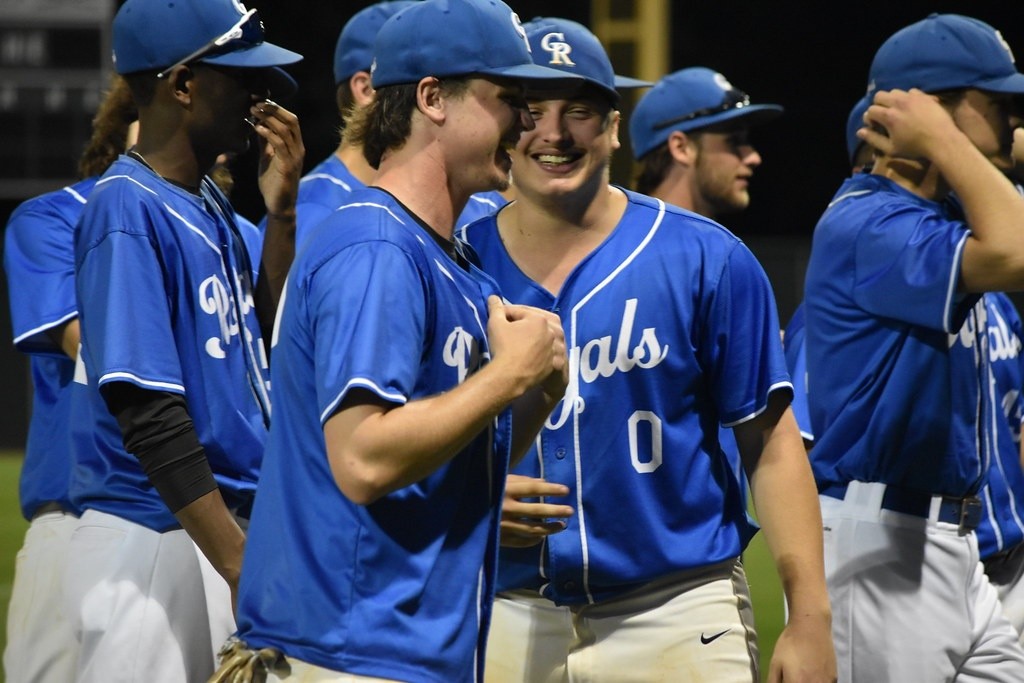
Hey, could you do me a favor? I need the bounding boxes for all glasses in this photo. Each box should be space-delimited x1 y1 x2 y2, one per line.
157 8 266 80
652 86 751 132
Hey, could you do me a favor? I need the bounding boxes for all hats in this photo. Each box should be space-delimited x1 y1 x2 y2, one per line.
111 0 305 76
332 0 413 81
846 95 877 164
627 67 782 163
864 12 1024 108
521 16 621 106
369 1 584 88
614 72 655 90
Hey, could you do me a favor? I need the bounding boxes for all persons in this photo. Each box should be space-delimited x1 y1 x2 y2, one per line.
454 17 838 683
235 0 569 683
630 65 784 683
2 73 260 682
64 0 304 683
258 0 426 366
784 14 1024 683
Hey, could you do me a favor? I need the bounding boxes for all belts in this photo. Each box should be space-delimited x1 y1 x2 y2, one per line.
30 501 65 522
235 505 252 521
821 481 986 535
